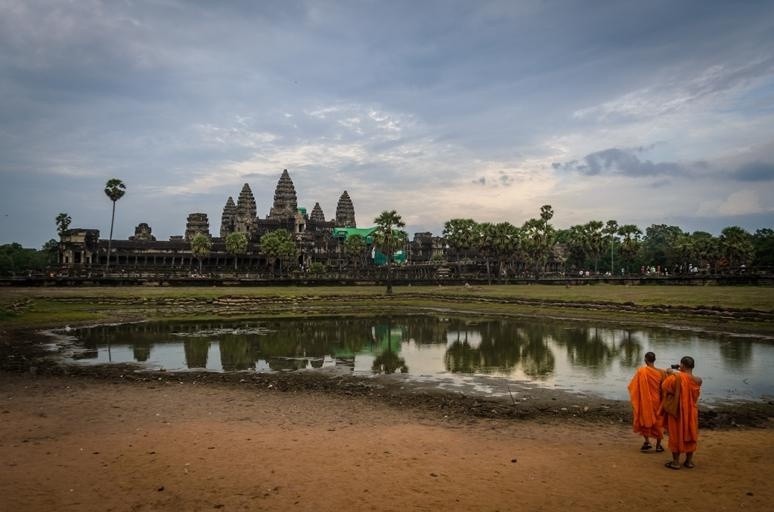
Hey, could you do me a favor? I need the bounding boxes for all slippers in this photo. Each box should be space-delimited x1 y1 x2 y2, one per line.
665 461 695 469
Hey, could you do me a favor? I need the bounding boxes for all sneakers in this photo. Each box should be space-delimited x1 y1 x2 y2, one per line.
641 442 664 452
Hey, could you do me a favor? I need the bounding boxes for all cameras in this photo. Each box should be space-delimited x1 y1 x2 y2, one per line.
671 365 678 369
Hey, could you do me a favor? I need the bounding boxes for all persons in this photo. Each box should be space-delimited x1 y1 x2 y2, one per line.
641 261 711 277
740 262 746 274
626 352 673 452
597 270 613 279
621 267 625 276
578 269 590 278
661 356 702 469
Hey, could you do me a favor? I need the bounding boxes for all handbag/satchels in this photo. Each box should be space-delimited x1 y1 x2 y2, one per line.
663 392 680 417
